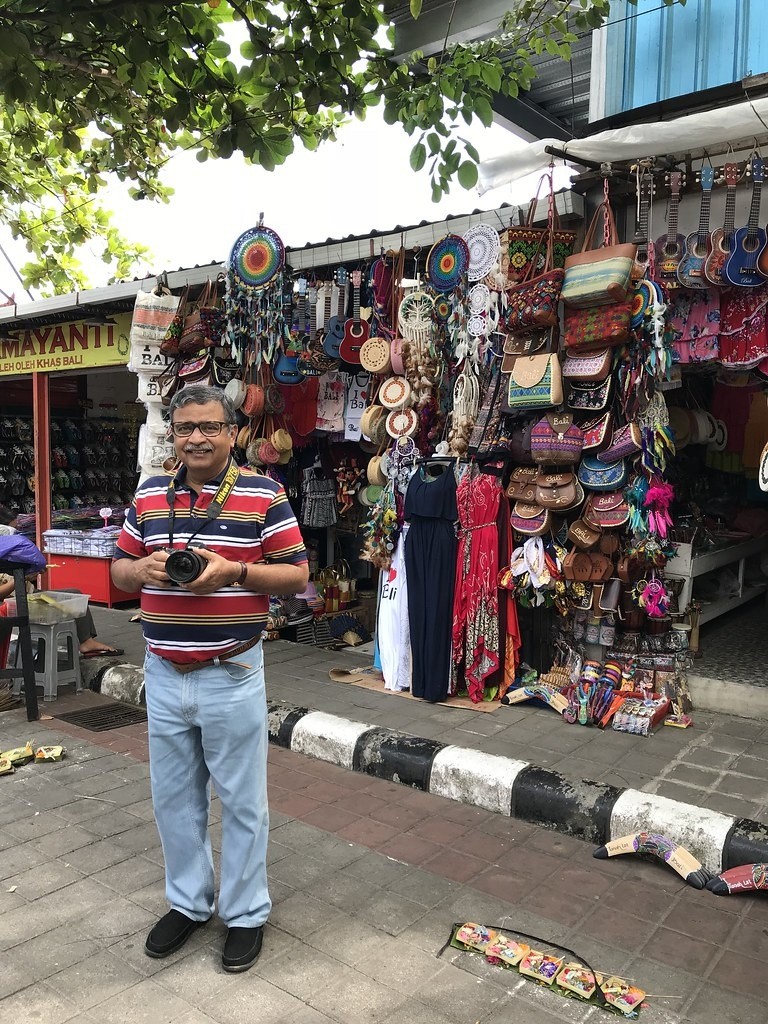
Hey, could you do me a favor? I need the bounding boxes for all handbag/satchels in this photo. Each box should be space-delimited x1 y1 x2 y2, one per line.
499 172 665 620
314 558 358 613
128 286 294 467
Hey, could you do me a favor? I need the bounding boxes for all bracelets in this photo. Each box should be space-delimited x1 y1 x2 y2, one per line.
231 561 247 587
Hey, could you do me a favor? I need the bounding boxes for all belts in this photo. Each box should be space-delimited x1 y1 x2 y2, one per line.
163 633 262 674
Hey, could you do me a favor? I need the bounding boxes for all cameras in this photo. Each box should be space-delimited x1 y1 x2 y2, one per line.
154 540 217 587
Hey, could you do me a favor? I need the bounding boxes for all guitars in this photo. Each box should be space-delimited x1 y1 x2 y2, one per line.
677 168 716 289
720 158 767 288
323 268 352 360
628 174 657 281
296 288 326 378
274 284 308 386
701 162 739 286
651 171 689 290
340 270 372 364
311 279 343 372
286 278 308 366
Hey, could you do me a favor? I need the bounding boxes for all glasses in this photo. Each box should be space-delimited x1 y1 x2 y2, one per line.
170 421 234 437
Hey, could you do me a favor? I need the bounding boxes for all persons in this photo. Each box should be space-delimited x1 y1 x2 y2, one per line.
0 504 125 669
110 384 309 972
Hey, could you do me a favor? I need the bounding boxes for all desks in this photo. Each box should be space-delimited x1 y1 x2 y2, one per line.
662 532 768 628
0 559 48 723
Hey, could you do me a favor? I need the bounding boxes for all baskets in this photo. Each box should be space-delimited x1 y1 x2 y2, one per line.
4 590 91 624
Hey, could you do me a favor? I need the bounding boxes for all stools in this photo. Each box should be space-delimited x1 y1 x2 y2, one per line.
11 619 83 702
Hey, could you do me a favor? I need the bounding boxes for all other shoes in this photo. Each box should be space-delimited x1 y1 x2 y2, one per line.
222 926 263 972
144 909 212 958
0 419 135 523
84 648 124 659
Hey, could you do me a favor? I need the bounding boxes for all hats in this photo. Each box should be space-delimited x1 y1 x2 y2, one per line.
283 581 326 625
